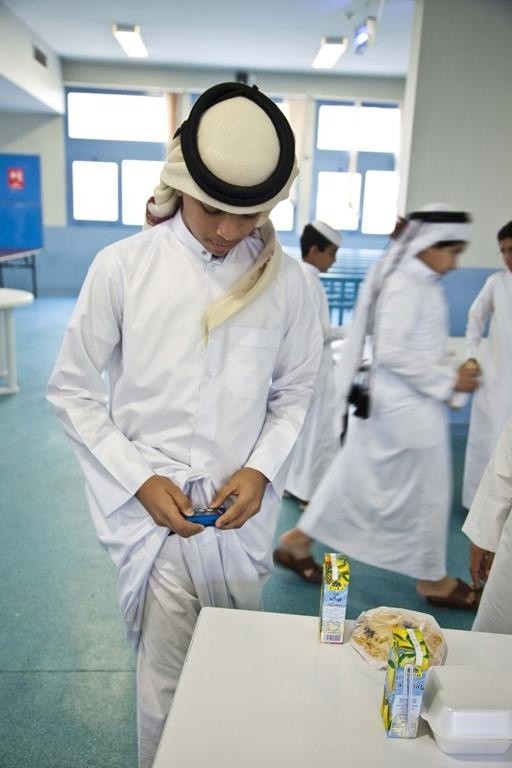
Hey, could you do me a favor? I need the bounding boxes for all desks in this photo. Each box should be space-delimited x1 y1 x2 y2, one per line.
321 263 374 325
0 248 42 298
151 607 512 768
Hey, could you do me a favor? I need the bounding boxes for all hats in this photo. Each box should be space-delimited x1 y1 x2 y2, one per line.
311 220 341 247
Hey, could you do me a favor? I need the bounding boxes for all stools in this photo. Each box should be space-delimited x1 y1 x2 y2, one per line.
2 288 34 397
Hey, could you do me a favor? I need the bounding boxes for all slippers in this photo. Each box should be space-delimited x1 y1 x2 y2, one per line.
272 549 323 584
425 578 483 608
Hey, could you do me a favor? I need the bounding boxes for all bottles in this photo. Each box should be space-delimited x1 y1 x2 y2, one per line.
450 357 478 407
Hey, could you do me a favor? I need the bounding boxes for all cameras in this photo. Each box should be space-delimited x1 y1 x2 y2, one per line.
187 507 226 527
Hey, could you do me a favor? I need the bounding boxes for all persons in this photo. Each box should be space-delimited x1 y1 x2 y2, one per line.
284 221 350 511
47 81 322 767
461 221 512 524
274 202 482 613
461 413 511 634
